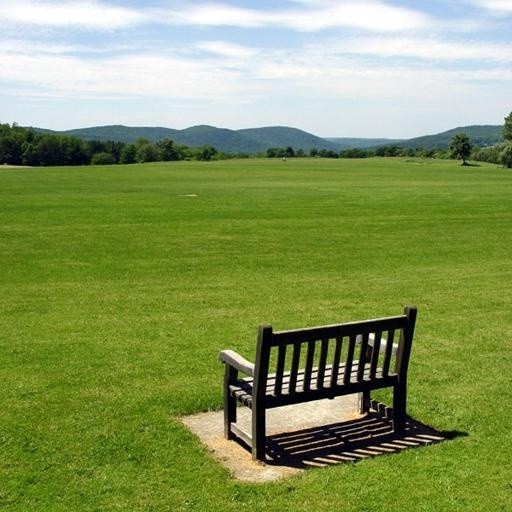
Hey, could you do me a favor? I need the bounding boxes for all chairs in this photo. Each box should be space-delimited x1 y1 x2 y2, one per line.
217 304 416 462
